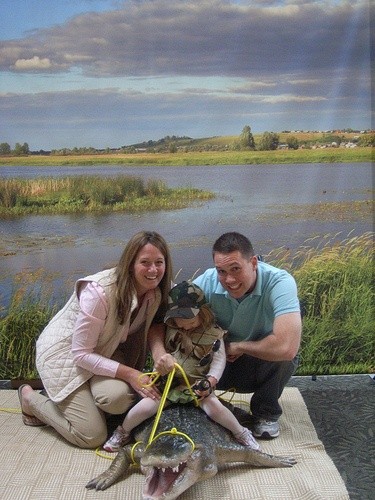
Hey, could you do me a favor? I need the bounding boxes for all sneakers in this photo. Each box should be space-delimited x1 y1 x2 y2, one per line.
103 425 131 452
233 427 260 451
253 414 281 439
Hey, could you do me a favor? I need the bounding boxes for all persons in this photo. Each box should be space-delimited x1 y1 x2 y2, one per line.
18 230 176 449
147 233 301 440
103 281 262 452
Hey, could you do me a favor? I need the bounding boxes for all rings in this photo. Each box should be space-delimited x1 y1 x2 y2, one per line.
141 388 144 391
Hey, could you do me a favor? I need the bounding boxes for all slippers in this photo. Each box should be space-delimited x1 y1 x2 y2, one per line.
17 383 46 426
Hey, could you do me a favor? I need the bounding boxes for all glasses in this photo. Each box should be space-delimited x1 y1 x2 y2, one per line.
198 339 220 366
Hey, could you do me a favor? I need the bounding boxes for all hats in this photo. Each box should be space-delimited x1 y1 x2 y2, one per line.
164 278 207 323
